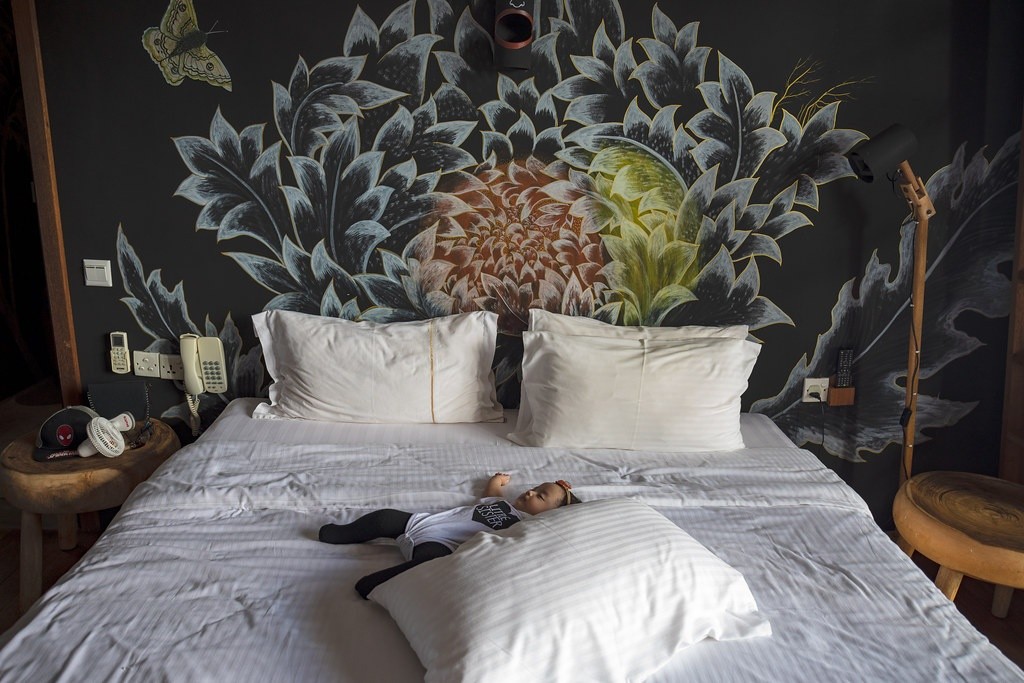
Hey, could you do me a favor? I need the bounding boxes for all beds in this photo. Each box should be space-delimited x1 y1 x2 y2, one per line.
0 392 1024 683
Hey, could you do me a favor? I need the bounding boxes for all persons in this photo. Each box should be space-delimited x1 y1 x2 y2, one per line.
318 471 583 600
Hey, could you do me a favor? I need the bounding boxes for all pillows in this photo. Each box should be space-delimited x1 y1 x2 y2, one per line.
506 305 761 455
250 308 506 425
367 494 773 683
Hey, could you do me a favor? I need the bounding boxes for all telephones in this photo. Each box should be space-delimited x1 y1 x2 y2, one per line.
178 333 227 395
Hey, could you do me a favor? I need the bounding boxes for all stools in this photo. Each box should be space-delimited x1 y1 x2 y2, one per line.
893 469 1023 623
0 414 181 604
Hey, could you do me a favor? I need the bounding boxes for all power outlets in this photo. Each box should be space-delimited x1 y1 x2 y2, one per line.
800 378 829 406
158 355 187 384
